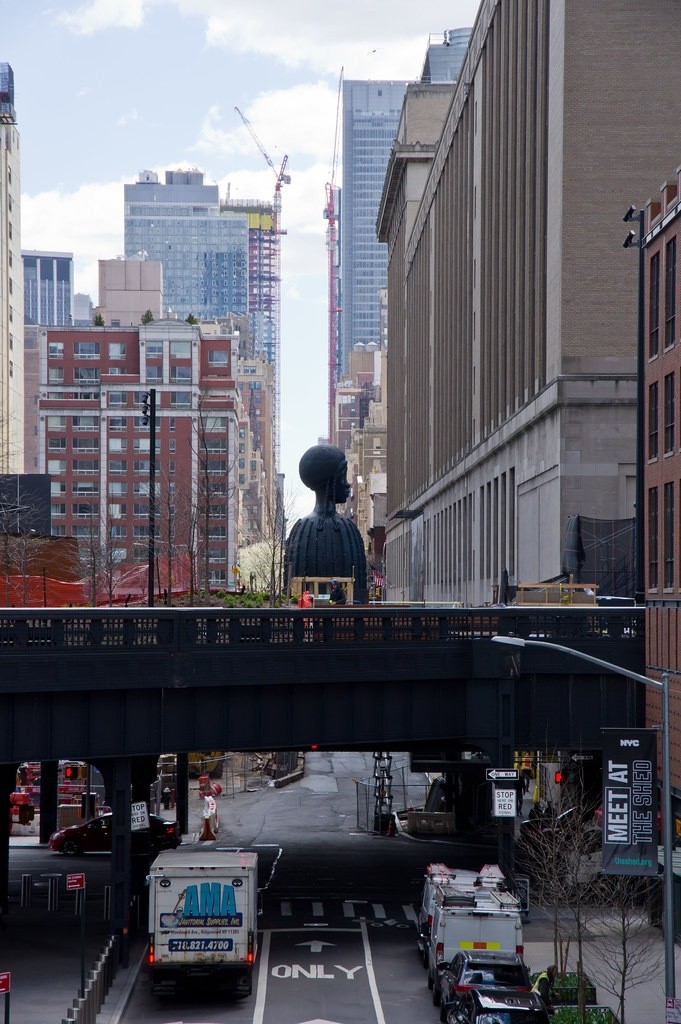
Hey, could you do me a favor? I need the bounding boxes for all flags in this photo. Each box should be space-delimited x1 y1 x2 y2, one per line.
373 570 384 587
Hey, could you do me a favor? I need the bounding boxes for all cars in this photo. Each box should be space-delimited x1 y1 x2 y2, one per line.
47 814 183 859
449 990 554 1024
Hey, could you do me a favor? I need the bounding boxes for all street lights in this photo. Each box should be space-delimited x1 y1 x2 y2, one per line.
141 387 155 607
491 634 677 997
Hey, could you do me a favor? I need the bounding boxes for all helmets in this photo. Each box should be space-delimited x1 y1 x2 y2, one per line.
332 580 339 589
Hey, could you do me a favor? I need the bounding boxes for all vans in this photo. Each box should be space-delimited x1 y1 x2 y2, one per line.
418 863 531 1007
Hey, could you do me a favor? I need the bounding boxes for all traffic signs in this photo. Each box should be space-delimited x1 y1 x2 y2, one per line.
486 768 519 781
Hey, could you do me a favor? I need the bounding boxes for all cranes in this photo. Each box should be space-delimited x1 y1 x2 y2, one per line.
323 64 347 443
234 106 291 475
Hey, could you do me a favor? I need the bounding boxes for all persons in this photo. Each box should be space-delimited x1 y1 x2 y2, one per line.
298 590 313 609
299 443 350 531
328 579 346 605
529 965 559 1008
528 801 557 820
515 752 538 816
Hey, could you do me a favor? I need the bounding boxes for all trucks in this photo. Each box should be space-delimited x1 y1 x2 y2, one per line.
145 851 263 997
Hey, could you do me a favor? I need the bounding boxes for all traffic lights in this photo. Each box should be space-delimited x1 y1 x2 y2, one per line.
555 771 563 785
65 766 80 779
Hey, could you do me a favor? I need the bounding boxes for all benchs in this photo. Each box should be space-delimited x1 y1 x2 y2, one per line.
313 603 416 641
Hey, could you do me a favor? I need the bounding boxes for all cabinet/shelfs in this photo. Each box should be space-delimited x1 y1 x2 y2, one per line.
516 574 599 606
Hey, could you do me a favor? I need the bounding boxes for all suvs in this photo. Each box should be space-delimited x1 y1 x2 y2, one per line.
438 949 533 1024
